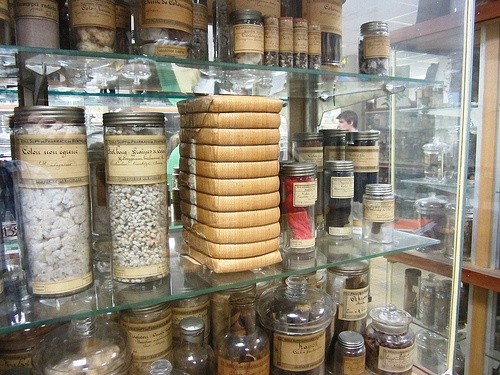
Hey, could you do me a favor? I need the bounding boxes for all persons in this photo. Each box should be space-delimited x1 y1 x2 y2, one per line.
166 132 181 229
336 111 358 131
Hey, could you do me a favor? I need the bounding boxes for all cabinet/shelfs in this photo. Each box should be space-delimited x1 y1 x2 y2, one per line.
382 0 500 374
0 0 476 374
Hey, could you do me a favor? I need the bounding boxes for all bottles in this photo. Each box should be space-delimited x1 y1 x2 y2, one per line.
211 0 347 72
0 0 209 62
450 0 466 13
10 106 95 300
102 112 172 285
0 252 417 375
279 128 394 254
443 50 478 102
87 149 111 263
358 20 390 77
403 124 477 375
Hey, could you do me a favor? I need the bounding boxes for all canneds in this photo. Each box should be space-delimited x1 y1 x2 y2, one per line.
226 8 322 71
102 111 171 284
13 105 93 298
278 128 379 253
356 21 390 76
